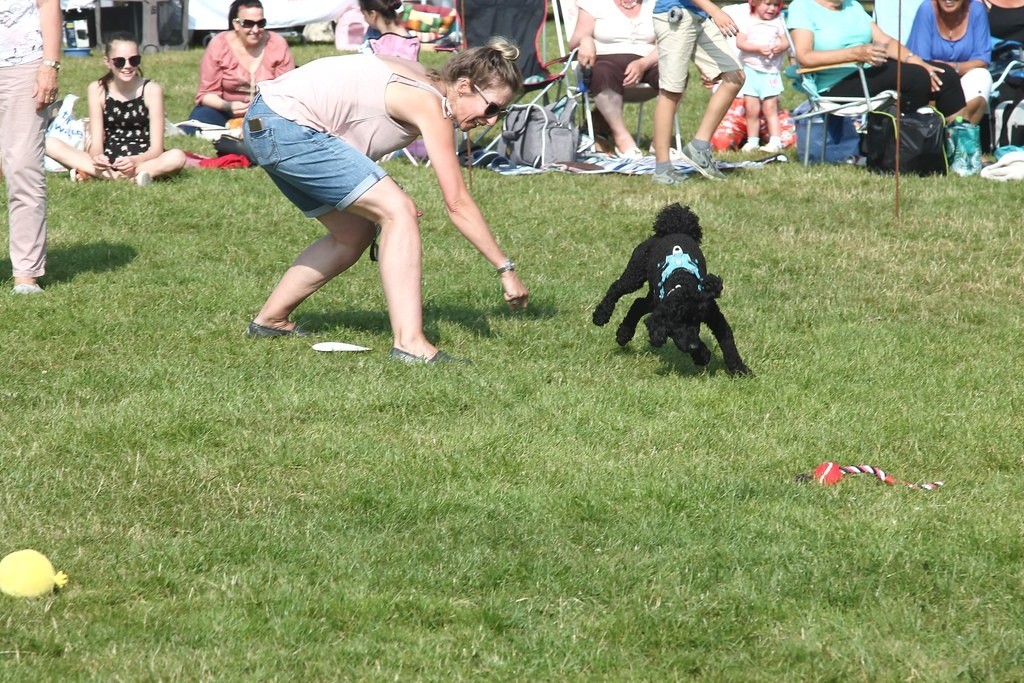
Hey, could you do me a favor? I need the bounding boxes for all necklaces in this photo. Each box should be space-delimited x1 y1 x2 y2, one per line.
621 0 637 9
939 13 955 39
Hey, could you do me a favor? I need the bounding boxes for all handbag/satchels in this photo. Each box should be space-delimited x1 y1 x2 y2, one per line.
858 106 950 175
943 116 985 177
213 135 258 166
497 95 580 168
45 94 83 172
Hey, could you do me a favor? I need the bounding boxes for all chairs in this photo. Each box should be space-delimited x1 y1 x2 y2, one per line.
433 0 996 169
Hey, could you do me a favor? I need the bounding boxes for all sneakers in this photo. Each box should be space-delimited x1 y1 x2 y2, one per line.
677 142 727 182
653 169 689 186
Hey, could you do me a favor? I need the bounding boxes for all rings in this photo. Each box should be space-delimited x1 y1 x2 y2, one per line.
930 72 936 76
635 80 639 84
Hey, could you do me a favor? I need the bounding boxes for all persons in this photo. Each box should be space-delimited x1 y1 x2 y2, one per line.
0 0 1024 364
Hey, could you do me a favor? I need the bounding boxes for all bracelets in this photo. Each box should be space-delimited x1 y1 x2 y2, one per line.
954 62 959 73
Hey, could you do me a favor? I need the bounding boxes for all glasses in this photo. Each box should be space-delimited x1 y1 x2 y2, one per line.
236 18 267 29
471 82 508 123
109 55 141 68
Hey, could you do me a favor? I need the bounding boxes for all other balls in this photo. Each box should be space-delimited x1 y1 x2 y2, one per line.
814 462 841 484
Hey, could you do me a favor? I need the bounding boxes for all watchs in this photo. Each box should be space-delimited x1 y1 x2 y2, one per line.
41 59 61 70
904 53 916 62
497 259 514 275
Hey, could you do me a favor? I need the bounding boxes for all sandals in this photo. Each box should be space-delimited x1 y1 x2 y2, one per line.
135 173 153 186
70 168 91 184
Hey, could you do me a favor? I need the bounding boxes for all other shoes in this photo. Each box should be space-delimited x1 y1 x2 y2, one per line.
390 346 471 367
14 283 45 293
461 149 499 167
608 143 643 161
760 141 783 153
246 320 312 337
742 143 760 152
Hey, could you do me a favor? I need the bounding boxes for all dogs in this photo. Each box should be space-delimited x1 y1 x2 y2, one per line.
590 199 753 380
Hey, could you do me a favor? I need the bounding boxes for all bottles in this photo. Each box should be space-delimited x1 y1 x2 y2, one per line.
955 116 962 126
